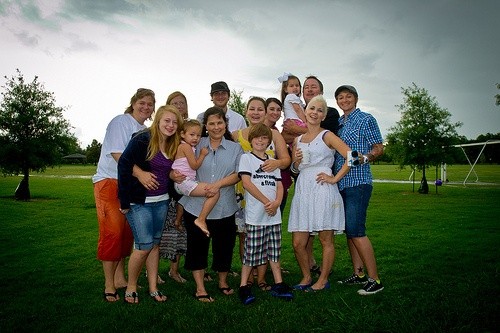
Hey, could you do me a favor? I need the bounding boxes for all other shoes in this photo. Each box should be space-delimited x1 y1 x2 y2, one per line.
168 271 187 283
146 270 165 284
318 270 333 277
309 266 320 276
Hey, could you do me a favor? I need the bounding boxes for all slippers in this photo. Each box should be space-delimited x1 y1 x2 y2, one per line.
197 295 214 302
150 290 166 304
219 285 234 296
125 292 138 304
105 290 120 302
257 283 272 292
203 272 213 282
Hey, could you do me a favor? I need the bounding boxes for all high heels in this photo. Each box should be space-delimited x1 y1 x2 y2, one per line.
304 280 330 292
292 279 315 290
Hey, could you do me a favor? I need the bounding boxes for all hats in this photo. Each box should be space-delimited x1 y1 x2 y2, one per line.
211 81 229 93
334 85 358 97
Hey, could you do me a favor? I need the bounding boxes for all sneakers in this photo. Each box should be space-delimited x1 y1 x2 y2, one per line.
272 281 294 300
240 285 255 304
337 274 368 285
357 279 384 295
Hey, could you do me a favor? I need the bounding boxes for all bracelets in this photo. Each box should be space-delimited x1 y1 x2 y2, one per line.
290 161 299 174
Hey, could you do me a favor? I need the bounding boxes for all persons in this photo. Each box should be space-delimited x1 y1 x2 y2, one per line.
92 88 160 303
118 72 354 305
333 85 384 296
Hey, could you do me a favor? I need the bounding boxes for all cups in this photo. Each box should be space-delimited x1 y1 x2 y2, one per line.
347 150 353 166
300 148 310 164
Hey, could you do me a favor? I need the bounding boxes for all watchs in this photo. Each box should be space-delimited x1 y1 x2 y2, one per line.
362 155 369 164
